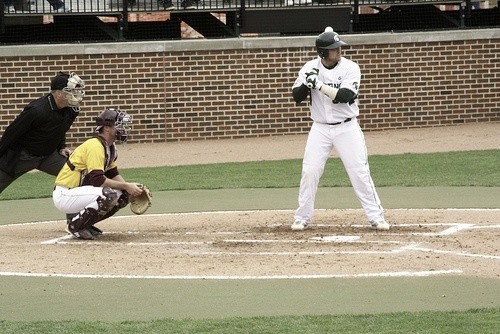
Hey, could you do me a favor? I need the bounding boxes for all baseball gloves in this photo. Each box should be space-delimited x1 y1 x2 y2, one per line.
129 185 151 216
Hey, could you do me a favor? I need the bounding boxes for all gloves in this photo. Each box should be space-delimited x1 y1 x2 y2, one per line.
304 68 324 92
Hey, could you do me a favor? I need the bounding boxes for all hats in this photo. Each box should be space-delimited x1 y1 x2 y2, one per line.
50 70 82 91
96 108 129 127
315 30 346 50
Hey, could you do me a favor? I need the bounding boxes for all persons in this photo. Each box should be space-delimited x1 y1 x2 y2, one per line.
291 32 389 230
53 108 143 240
0 70 85 194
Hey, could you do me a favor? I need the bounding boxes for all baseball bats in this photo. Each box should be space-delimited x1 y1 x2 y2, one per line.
307 25 335 88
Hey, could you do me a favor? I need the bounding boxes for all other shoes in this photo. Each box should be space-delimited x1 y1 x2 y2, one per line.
64 222 104 240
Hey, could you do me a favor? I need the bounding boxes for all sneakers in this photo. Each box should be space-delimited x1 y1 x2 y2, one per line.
291 219 310 232
369 219 392 231
